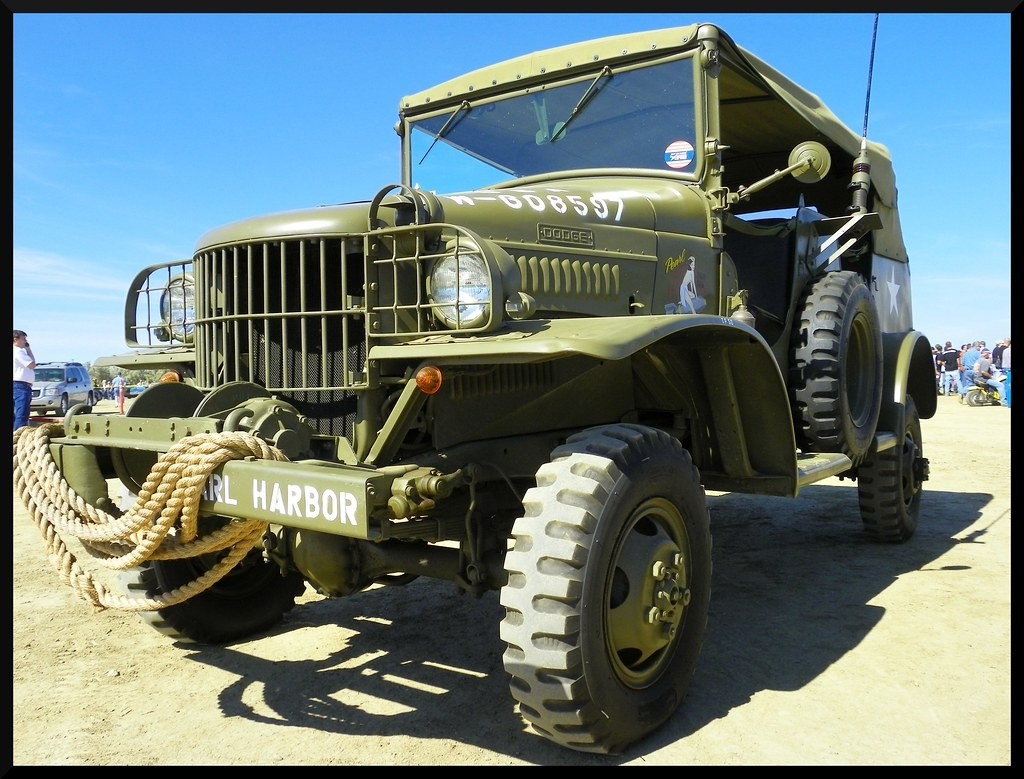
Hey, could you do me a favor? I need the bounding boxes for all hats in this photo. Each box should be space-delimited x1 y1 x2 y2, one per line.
980 347 992 354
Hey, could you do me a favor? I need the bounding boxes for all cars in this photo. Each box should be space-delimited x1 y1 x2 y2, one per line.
94 388 102 406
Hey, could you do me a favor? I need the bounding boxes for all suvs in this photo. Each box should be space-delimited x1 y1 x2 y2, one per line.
15 13 937 754
29 362 95 415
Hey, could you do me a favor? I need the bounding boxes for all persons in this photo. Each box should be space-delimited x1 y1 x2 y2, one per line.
14 330 36 430
93 371 148 407
931 337 1010 407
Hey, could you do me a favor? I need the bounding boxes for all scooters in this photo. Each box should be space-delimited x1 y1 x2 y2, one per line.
966 375 1007 407
